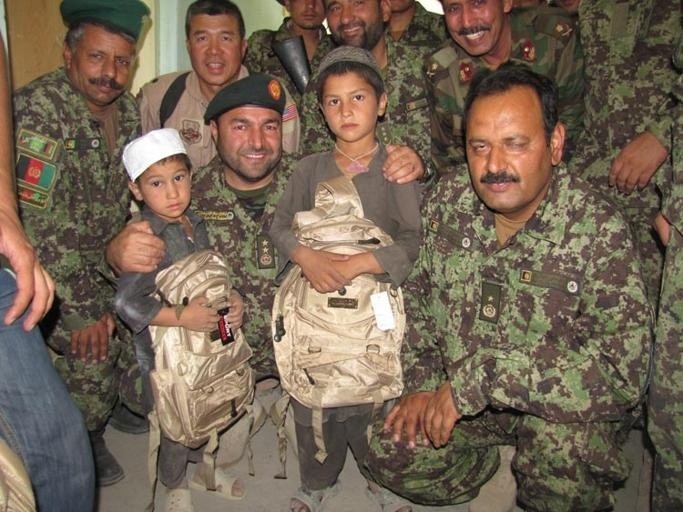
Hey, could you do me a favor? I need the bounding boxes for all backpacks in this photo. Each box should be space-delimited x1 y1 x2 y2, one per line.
269 176 409 409
141 248 259 454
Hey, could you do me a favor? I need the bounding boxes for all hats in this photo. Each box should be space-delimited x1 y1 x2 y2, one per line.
202 71 289 118
313 43 385 79
57 1 151 35
121 126 189 184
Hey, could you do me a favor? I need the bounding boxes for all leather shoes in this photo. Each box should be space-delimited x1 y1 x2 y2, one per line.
83 431 125 486
108 402 150 434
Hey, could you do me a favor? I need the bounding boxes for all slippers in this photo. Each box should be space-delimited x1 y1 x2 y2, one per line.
363 480 415 512
189 466 248 500
290 473 341 511
161 484 195 512
